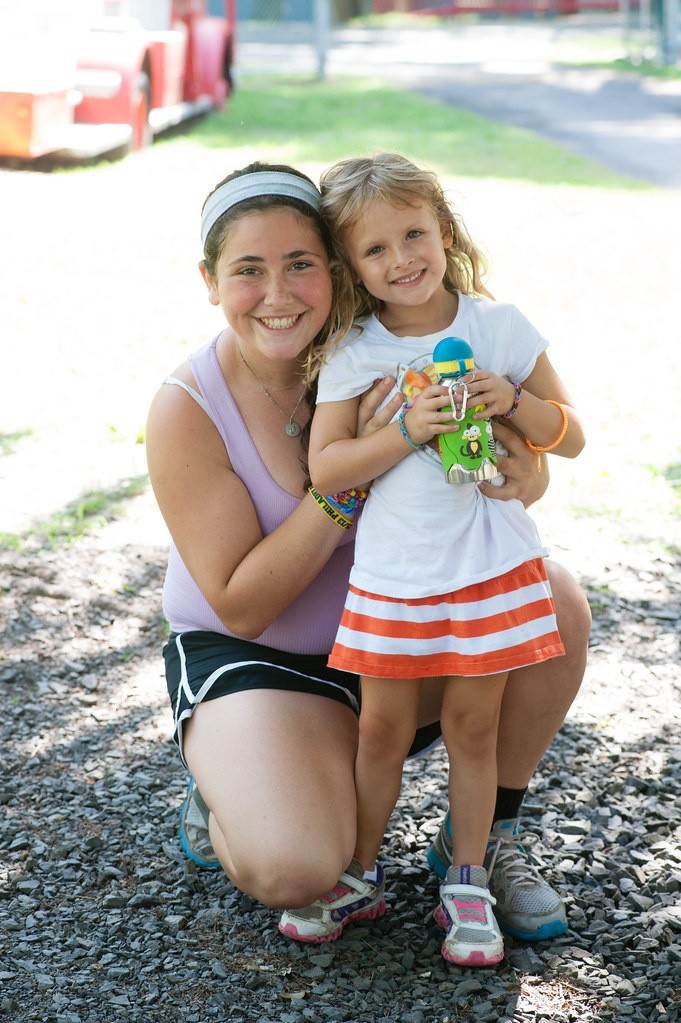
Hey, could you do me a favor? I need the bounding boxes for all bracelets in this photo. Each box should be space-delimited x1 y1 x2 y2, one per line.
525 400 568 475
500 378 523 418
398 406 423 452
304 471 371 532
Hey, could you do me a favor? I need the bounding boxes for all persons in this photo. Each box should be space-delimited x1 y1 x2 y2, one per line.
143 160 593 943
274 151 587 970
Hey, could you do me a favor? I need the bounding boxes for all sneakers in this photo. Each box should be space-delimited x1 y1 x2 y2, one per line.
276 856 388 944
428 805 569 941
430 865 505 970
181 775 224 871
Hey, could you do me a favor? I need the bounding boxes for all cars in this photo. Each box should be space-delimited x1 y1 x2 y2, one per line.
0 0 233 162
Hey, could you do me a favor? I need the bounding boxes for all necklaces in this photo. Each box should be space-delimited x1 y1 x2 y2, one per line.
232 329 317 438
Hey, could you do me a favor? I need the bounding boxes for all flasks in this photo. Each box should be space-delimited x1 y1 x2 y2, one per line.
431 335 500 485
